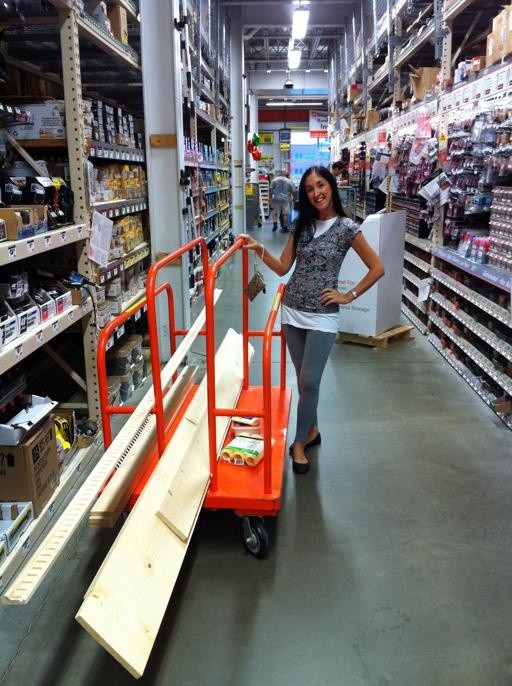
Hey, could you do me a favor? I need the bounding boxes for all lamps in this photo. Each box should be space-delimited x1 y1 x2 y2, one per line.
292 7 309 40
266 102 323 106
289 49 301 70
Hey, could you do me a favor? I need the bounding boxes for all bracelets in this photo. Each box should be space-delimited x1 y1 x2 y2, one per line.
350 290 358 298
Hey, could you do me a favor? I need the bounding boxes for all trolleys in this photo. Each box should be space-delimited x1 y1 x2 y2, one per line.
96 236 292 558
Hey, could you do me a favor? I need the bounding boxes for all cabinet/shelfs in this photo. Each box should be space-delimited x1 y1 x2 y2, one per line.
178 3 246 337
328 1 512 431
1 0 190 653
259 180 269 217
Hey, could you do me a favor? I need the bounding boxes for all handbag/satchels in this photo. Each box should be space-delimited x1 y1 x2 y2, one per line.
241 243 267 302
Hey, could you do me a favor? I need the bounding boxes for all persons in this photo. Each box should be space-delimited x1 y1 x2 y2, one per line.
269 168 297 233
330 162 348 186
236 167 384 474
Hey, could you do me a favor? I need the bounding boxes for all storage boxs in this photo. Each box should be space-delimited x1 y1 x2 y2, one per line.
133 359 144 385
120 376 133 402
107 376 121 416
487 33 493 67
116 334 143 362
412 67 440 101
337 210 408 337
0 413 59 518
106 343 133 376
493 5 512 63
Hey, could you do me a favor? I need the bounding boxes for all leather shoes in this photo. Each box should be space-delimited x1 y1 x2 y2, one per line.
289 432 321 453
291 448 311 475
